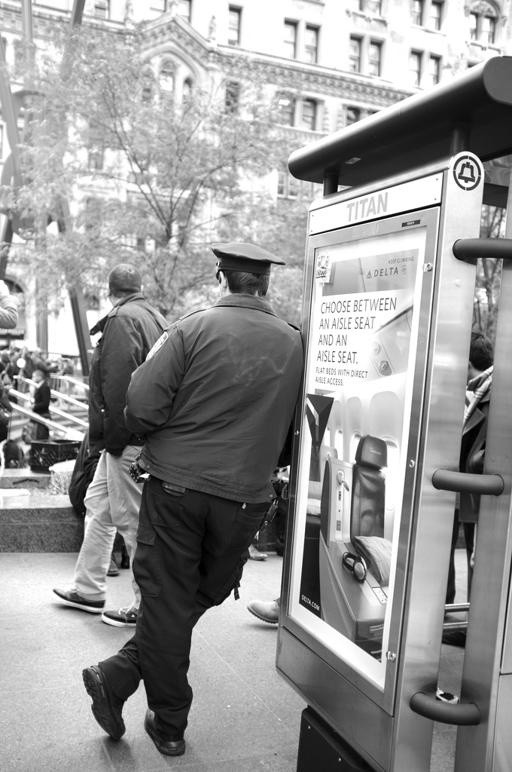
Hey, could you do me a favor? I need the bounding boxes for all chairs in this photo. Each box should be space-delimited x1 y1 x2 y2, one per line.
349 435 389 544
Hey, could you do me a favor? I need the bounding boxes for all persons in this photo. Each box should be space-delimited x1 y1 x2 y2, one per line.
437 332 494 604
53 264 172 628
246 595 281 625
0 345 75 469
0 279 20 330
79 241 316 759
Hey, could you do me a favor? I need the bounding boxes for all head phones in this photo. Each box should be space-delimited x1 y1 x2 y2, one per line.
340 551 368 583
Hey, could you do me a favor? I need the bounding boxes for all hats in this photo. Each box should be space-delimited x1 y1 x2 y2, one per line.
211 243 286 280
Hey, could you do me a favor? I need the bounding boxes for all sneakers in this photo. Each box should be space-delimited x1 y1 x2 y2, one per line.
102 606 137 627
52 588 105 613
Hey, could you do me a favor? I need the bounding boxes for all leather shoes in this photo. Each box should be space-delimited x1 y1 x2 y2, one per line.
83 665 125 739
248 598 281 624
106 555 120 576
145 709 185 755
248 545 268 559
